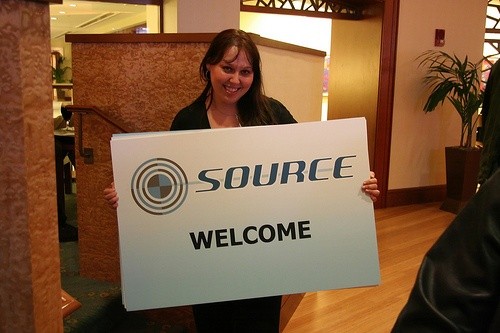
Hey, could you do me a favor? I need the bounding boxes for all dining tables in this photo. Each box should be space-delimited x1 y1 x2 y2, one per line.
54 126 76 241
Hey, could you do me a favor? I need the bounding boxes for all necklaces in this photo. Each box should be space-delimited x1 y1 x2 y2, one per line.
213 103 243 129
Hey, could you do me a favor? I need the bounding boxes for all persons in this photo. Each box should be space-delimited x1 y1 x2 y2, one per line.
102 29 381 333
476 57 500 188
390 168 500 333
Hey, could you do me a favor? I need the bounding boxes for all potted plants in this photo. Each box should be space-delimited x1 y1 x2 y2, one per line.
415 49 498 216
52 67 70 99
